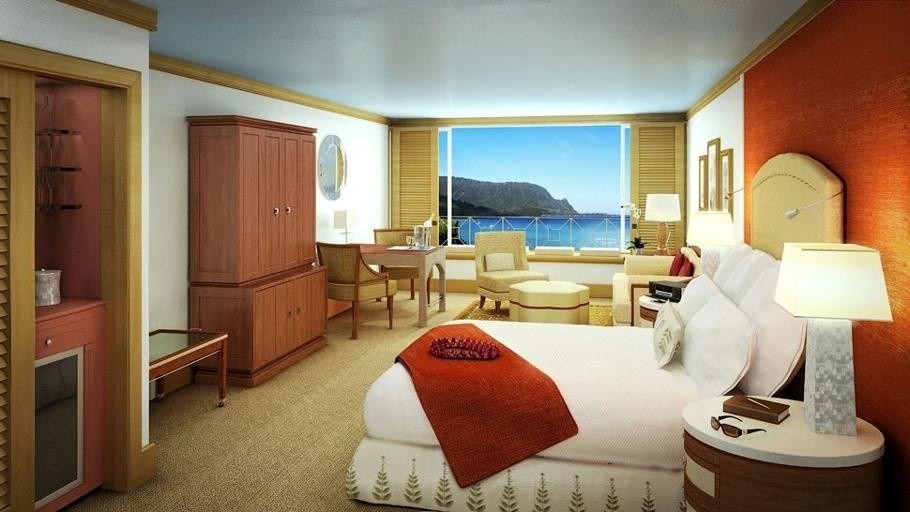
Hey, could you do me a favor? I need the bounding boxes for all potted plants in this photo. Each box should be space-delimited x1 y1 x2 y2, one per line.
625 236 648 256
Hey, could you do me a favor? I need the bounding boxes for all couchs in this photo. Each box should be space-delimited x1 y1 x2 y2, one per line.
613 255 699 326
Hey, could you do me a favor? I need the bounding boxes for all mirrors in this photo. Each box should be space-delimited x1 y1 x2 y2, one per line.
317 134 347 201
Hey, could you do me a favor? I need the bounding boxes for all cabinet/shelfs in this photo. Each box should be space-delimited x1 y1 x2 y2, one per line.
185 116 327 388
34 300 112 512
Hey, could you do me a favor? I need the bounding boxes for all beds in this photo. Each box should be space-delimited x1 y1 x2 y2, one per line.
345 153 844 512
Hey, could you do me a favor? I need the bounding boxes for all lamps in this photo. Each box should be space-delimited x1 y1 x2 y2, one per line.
774 244 893 436
785 192 842 218
724 187 744 199
644 193 681 255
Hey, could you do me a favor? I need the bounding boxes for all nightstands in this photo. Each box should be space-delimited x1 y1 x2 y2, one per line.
681 397 884 512
639 295 681 328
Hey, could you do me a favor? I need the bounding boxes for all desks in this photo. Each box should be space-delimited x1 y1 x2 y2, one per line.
360 244 446 329
150 330 228 407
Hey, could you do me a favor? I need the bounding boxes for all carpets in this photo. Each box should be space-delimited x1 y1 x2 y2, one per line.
453 300 614 327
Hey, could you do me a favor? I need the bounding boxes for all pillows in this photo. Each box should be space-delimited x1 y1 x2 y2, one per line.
485 253 515 271
652 244 807 396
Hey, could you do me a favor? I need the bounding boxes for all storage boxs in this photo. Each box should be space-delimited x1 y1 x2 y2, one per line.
649 281 687 302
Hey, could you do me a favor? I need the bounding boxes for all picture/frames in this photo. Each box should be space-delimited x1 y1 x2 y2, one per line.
699 138 734 214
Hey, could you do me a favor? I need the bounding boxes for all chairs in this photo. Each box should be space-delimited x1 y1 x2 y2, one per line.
475 231 548 312
316 242 397 339
373 229 433 304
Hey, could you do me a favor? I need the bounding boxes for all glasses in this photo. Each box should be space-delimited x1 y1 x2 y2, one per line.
711 414 766 437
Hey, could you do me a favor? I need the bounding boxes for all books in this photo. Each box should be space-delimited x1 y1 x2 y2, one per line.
722 395 792 425
